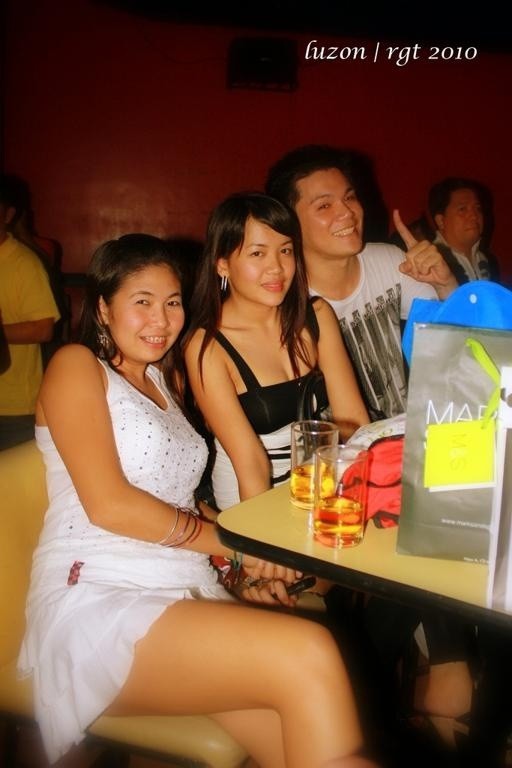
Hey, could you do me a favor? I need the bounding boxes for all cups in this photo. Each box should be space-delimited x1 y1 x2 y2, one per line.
312 444 370 548
288 420 338 509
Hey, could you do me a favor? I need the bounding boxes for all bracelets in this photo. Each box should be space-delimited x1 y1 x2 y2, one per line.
156 503 179 542
168 513 197 548
177 517 202 544
164 507 191 547
230 547 244 584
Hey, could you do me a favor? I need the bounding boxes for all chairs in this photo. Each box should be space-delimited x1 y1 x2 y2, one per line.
0 440 251 767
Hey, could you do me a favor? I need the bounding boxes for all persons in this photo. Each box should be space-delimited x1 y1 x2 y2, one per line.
421 174 500 286
180 192 478 750
0 166 61 452
261 143 458 425
18 234 364 768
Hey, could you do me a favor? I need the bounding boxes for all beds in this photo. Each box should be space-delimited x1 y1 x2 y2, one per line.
215 479 512 768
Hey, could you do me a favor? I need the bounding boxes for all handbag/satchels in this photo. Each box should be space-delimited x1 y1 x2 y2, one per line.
393 325 512 561
